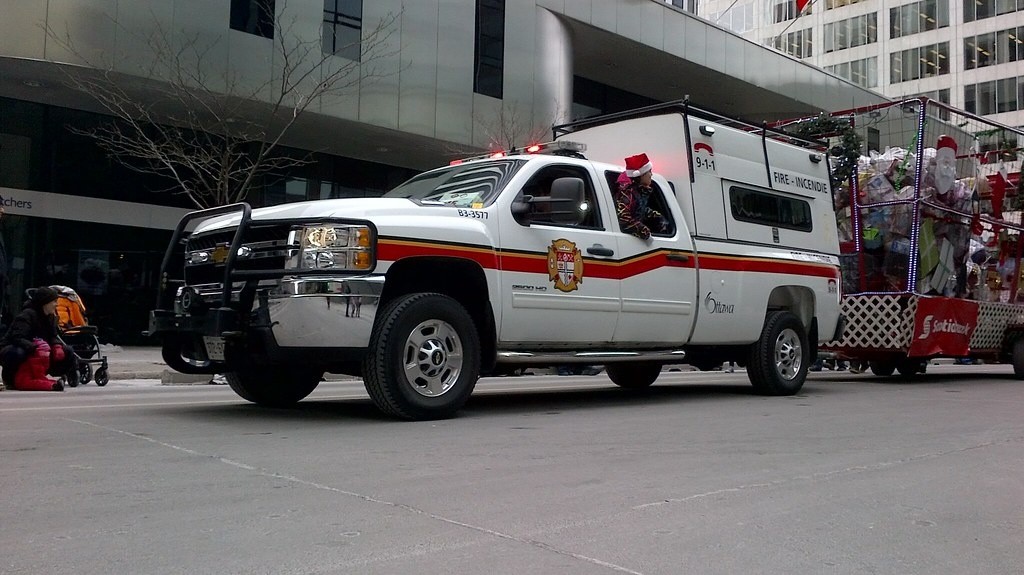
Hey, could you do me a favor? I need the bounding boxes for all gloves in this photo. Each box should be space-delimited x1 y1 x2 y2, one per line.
640 226 650 240
35 348 46 357
51 344 64 360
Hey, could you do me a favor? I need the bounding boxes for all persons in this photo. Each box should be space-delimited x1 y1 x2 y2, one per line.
326 293 331 310
615 154 672 246
344 286 362 317
13 338 64 391
0 288 65 391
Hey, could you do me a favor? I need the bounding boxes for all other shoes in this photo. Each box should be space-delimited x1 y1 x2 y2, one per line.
713 366 722 371
558 371 573 375
346 315 349 317
809 366 822 372
823 362 834 370
850 368 859 374
725 366 734 373
837 367 846 371
859 366 869 373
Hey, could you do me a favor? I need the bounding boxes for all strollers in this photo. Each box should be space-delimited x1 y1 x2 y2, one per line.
25 284 109 387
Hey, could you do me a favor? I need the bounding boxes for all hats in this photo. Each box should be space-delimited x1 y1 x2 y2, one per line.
33 338 51 351
625 153 651 177
34 287 59 305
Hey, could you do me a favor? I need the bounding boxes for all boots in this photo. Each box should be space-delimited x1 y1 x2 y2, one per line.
52 375 67 391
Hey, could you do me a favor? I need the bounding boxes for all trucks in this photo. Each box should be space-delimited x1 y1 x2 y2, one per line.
140 95 841 420
